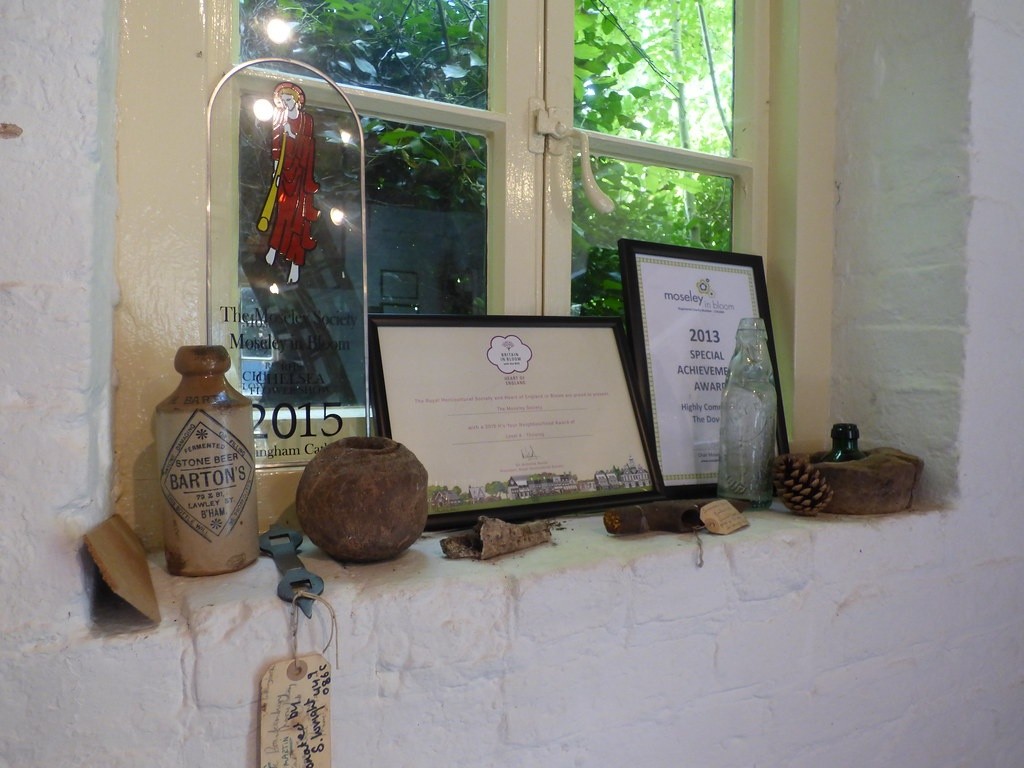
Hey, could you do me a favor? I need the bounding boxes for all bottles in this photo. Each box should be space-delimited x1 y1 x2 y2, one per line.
820 423 867 464
717 318 777 511
152 345 259 575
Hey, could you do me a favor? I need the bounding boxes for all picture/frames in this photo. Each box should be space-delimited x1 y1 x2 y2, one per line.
618 239 798 502
367 316 662 530
